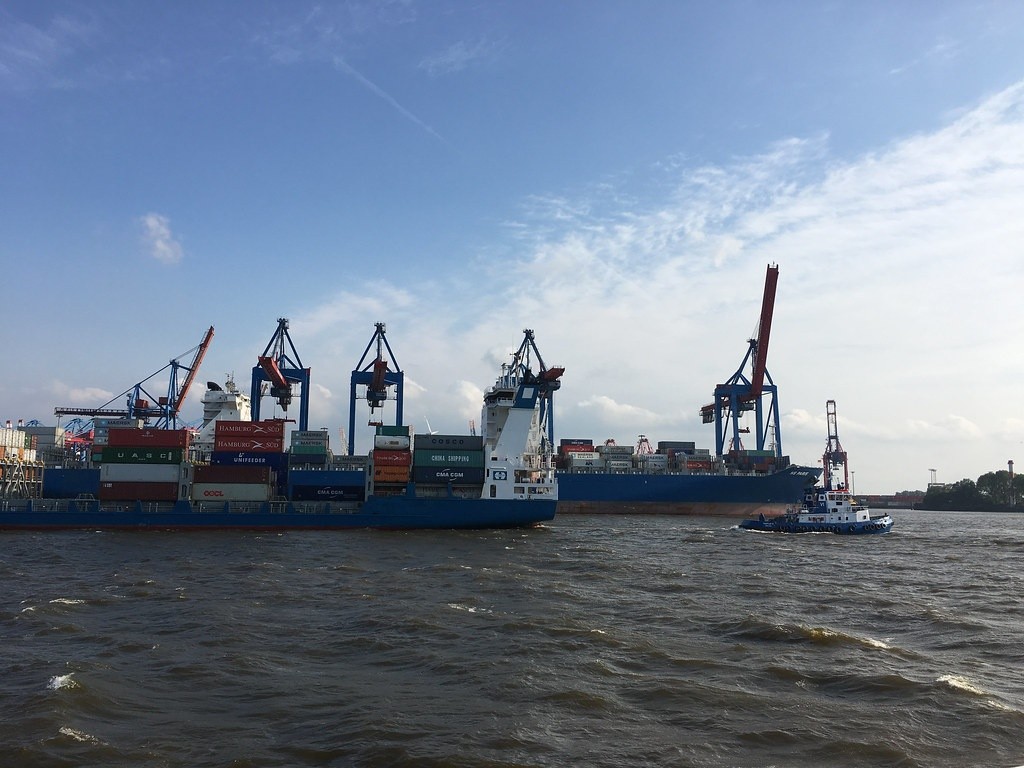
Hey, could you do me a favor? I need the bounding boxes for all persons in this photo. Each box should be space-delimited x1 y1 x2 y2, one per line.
0 453 21 478
759 512 766 521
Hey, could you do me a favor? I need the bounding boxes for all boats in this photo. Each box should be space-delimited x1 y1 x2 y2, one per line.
739 490 894 534
560 264 823 516
0 318 565 531
